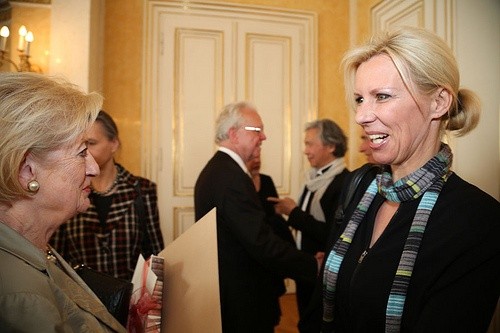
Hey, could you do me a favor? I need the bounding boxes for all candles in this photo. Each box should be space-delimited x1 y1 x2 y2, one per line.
0 26 9 51
18 25 27 51
25 32 32 55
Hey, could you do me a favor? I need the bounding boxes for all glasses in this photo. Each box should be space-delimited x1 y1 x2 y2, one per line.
237 127 263 133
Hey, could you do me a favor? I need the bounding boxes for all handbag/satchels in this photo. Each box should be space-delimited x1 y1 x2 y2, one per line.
73 267 132 326
